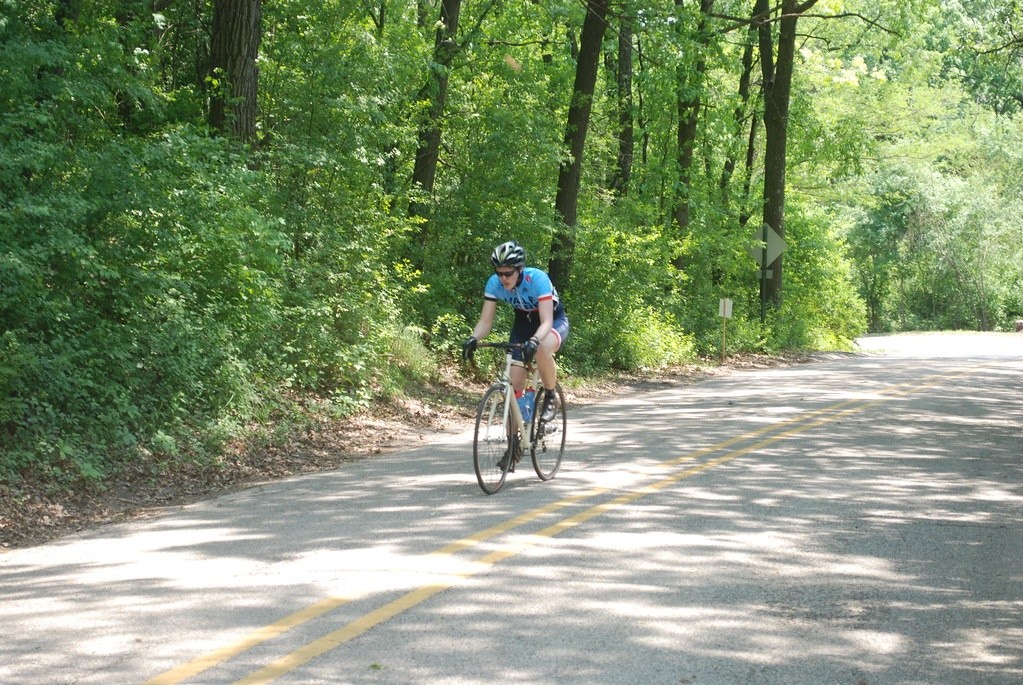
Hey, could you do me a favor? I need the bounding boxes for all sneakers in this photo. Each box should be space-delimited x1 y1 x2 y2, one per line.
496 450 514 472
540 395 557 421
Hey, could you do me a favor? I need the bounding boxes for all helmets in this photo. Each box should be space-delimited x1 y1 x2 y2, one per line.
490 240 526 268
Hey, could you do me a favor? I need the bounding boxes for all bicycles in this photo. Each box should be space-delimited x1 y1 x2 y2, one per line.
462 338 568 496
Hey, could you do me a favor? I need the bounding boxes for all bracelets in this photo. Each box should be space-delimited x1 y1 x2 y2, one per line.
530 335 541 347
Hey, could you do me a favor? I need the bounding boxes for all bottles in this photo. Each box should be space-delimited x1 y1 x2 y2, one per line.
524 386 535 420
514 389 530 421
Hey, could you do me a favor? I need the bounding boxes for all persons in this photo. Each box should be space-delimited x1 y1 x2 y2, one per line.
462 242 569 468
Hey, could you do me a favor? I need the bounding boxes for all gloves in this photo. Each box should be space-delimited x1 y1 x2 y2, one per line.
518 335 540 362
461 336 478 360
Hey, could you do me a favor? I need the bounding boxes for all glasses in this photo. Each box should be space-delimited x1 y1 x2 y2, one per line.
494 267 517 277
